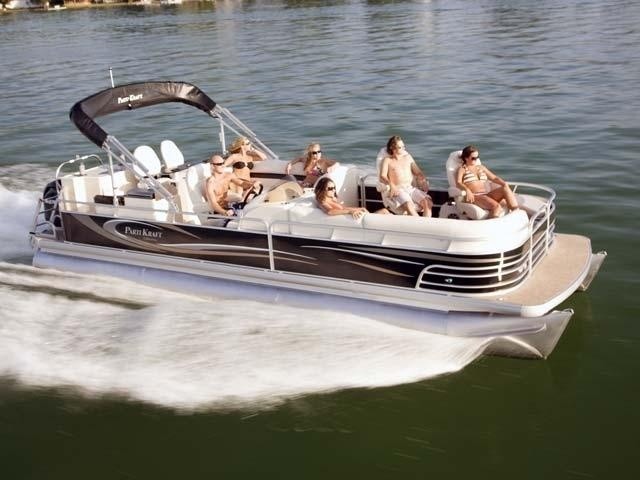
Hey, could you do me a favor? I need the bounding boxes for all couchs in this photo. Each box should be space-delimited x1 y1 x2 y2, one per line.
289 197 527 237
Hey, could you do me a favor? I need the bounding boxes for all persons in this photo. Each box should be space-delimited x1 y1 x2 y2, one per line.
315 177 390 219
285 142 339 189
204 136 261 215
380 136 433 217
455 147 518 219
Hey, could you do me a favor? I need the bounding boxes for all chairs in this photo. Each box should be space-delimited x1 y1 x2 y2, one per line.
185 164 242 226
377 147 431 215
446 150 504 220
130 140 191 191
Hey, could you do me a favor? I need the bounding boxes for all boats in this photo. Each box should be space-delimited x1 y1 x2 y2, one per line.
28 66 608 363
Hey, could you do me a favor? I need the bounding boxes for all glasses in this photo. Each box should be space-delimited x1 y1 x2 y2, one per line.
313 151 322 154
472 157 479 160
246 142 250 145
213 162 224 165
328 187 335 190
